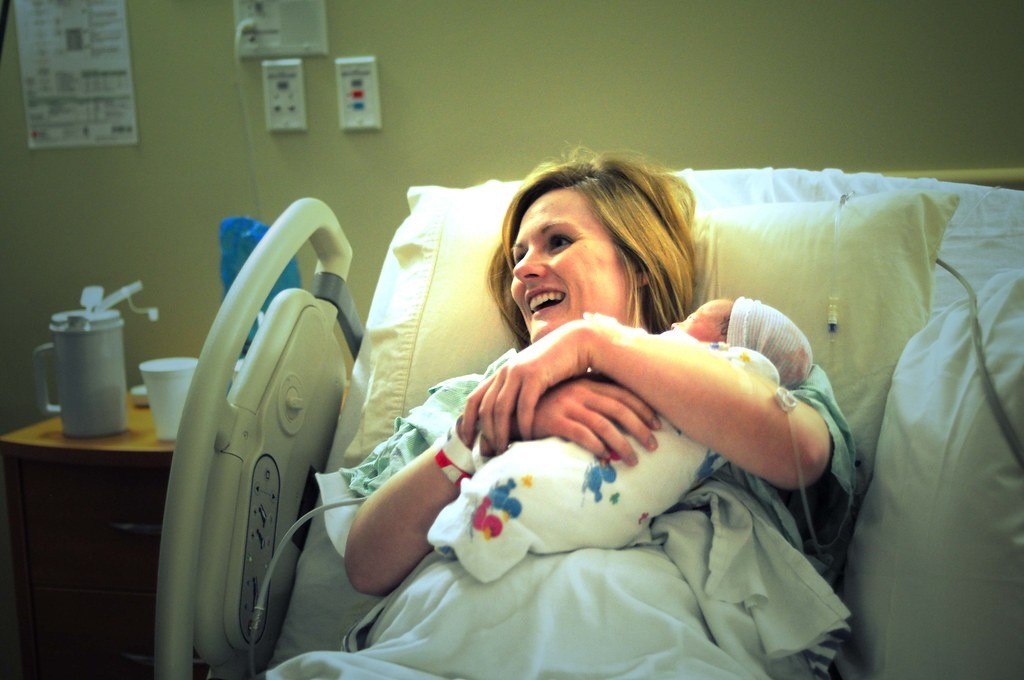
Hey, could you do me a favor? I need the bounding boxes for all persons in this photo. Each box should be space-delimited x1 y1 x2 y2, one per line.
344 140 858 680
426 295 814 584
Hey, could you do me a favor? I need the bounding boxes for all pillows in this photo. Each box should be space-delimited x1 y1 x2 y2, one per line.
343 167 963 497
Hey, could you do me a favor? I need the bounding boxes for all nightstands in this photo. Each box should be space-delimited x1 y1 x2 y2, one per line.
0 389 180 680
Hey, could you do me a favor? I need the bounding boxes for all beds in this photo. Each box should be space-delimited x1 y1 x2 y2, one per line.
157 167 1024 679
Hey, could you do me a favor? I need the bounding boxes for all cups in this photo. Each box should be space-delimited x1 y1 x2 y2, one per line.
32 310 128 438
137 357 198 441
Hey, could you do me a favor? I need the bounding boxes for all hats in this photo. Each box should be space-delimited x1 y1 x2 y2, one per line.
727 296 812 389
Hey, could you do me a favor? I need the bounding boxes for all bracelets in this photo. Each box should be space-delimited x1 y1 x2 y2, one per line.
434 414 483 488
